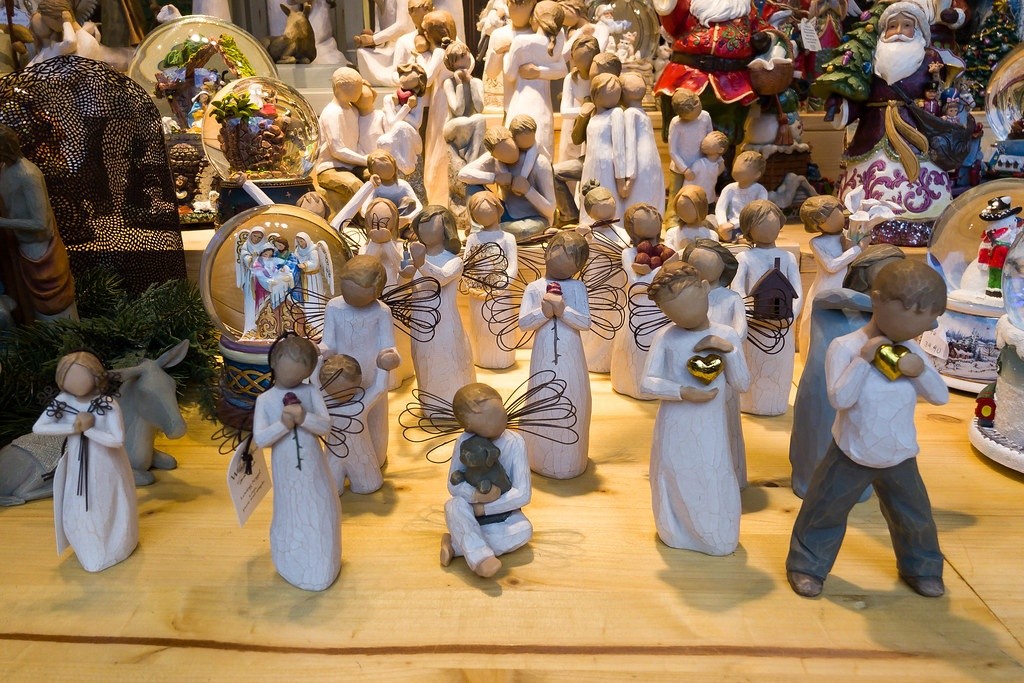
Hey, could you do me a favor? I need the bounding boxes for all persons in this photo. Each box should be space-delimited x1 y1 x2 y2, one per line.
0 0 974 595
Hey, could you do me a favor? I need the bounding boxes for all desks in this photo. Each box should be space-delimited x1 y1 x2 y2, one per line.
0 348 1024 683
62 223 928 335
